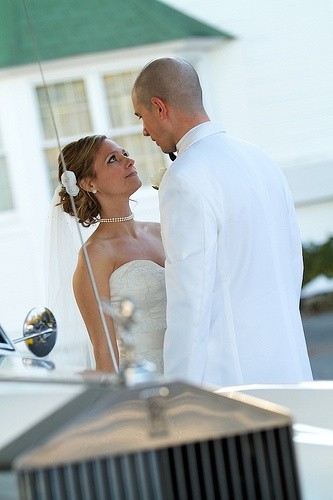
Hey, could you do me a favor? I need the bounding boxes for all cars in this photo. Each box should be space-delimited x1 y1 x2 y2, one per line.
0 306 333 499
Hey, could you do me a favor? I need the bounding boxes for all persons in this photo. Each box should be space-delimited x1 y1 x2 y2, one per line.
53 135 167 373
131 56 313 387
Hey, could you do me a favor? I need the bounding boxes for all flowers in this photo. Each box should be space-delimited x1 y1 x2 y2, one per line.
61 171 79 196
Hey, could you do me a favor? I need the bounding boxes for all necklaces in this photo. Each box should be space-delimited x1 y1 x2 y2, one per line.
98 213 135 223
